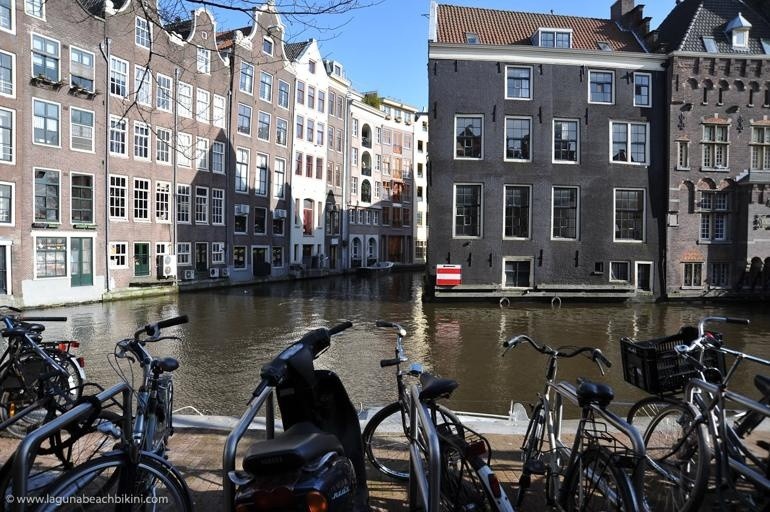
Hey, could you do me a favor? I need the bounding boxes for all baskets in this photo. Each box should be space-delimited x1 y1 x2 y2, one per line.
620 326 727 395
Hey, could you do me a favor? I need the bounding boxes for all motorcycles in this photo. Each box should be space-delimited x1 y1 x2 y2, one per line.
228 320 374 512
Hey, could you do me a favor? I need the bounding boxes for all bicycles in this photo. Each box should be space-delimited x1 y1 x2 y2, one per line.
362 319 515 512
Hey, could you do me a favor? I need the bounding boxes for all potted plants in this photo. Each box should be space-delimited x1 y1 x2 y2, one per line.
182 269 195 280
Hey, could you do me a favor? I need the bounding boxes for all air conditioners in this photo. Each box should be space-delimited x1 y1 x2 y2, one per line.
159 254 177 278
209 268 230 278
